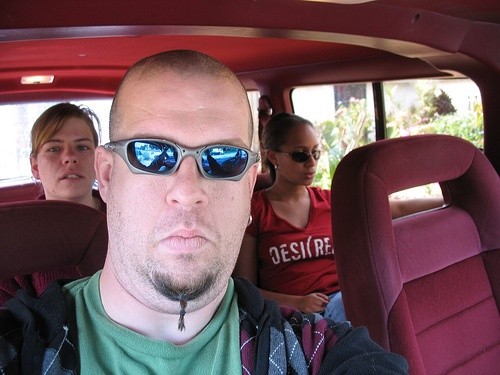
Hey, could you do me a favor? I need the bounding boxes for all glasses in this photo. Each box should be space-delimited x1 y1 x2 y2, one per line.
104 138 260 182
279 150 321 163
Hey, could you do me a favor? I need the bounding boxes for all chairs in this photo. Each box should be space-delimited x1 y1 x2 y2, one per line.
330 134 500 375
0 200 108 300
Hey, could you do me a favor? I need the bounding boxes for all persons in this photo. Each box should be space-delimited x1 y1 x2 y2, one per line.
232 113 445 323
0 48 410 375
1 102 106 308
